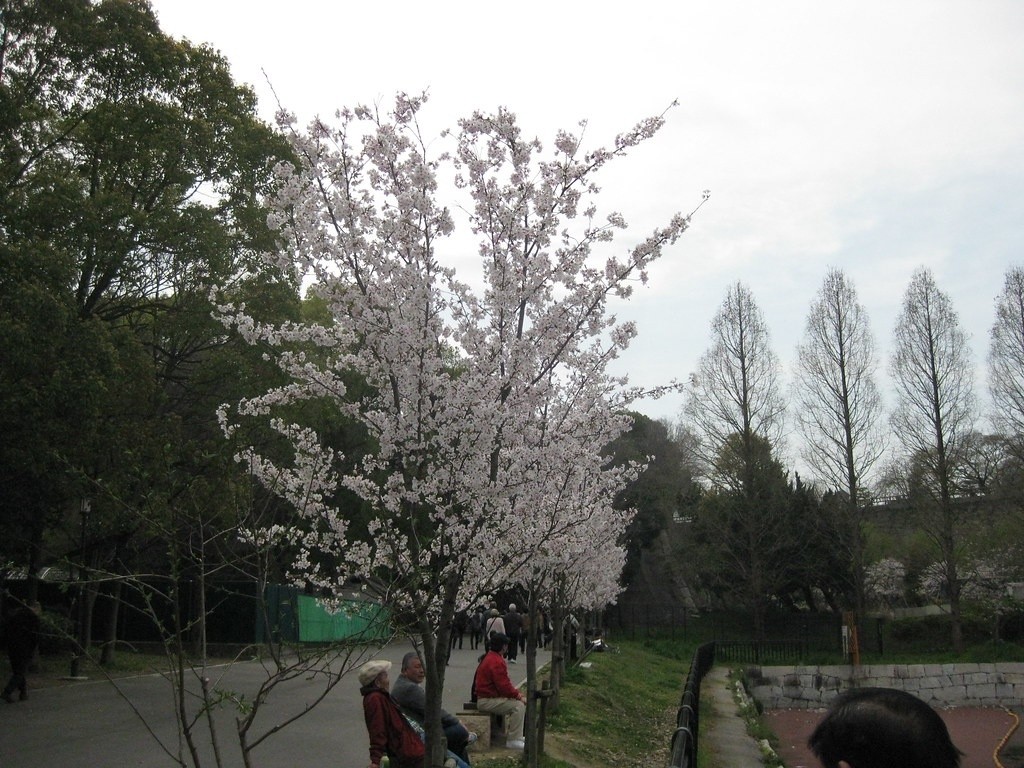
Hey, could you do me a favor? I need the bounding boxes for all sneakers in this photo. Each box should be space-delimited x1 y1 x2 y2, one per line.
466 732 477 745
506 736 525 749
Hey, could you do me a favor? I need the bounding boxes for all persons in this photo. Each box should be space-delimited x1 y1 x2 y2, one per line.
391 653 476 765
358 661 470 768
0 599 41 702
807 687 964 768
446 602 580 666
471 634 526 749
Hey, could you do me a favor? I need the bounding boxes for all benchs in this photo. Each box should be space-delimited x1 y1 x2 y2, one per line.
452 702 505 749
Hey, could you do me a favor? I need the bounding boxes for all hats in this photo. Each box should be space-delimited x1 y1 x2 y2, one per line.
358 659 392 686
491 609 498 615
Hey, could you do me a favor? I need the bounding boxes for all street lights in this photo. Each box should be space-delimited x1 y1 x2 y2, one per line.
72 495 92 655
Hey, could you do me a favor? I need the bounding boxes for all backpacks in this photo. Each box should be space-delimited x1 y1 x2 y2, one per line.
563 616 577 637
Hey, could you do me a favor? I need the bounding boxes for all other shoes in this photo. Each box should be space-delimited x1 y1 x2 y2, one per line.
511 659 517 664
